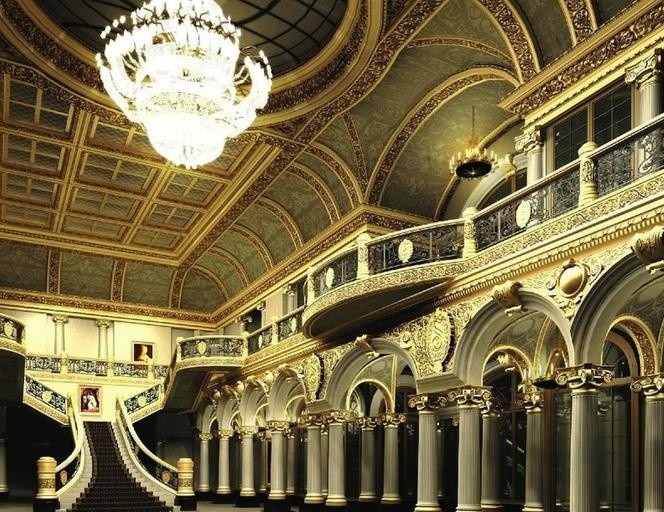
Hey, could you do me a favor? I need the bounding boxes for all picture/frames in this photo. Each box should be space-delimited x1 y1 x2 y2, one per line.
78 384 102 415
131 341 155 364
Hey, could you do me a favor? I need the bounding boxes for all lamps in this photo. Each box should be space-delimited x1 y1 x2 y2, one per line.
96 0 273 168
449 104 498 180
